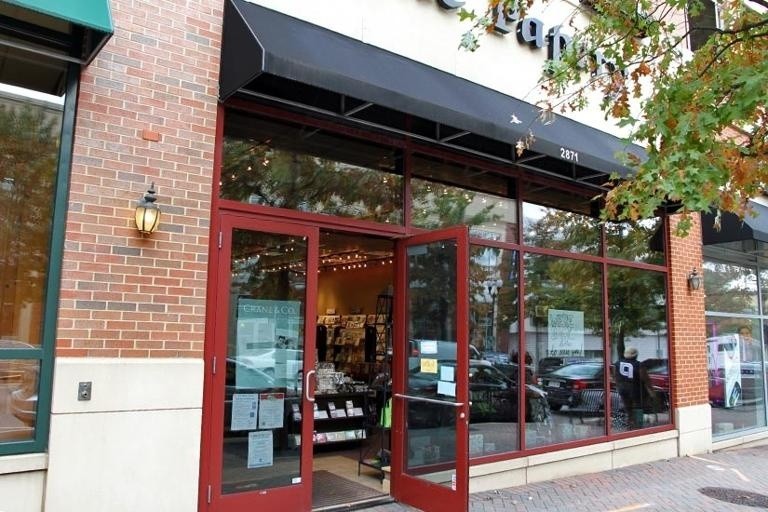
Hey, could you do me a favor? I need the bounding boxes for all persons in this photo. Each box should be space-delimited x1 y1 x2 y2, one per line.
469 335 486 359
614 346 657 431
732 324 758 431
275 335 297 364
512 350 533 366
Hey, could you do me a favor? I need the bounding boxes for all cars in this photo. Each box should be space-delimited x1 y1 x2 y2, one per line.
708 361 768 408
404 338 671 422
0 339 41 424
223 348 303 439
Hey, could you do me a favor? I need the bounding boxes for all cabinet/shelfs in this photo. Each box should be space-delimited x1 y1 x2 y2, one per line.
285 312 394 483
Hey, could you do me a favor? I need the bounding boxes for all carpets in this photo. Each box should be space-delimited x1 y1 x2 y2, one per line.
221 467 386 506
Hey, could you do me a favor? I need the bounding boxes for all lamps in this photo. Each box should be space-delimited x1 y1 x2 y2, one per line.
686 266 701 290
133 180 162 236
